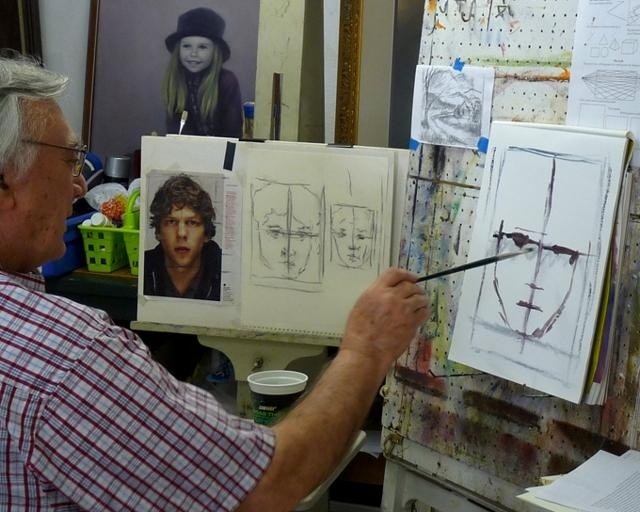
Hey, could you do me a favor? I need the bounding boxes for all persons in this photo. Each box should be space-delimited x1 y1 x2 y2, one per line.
144 173 223 301
1 57 432 511
163 7 241 136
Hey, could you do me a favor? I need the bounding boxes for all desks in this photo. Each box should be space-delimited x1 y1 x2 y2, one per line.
45 265 138 329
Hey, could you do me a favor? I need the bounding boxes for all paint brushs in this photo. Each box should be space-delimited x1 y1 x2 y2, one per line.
417 248 534 283
178 111 189 134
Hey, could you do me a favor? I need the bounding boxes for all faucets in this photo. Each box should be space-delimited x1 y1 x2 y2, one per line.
250 357 263 372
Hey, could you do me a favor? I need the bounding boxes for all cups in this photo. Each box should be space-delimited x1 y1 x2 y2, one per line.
246 370 308 427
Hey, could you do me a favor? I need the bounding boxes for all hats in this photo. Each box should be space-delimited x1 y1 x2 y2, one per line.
164 8 231 63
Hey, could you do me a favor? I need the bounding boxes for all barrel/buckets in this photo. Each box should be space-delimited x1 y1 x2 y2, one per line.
246 370 309 426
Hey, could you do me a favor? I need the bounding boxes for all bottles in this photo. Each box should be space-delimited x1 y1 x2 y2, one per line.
90 213 117 229
103 155 131 189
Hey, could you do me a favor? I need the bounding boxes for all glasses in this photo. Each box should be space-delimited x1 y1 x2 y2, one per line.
19 141 88 177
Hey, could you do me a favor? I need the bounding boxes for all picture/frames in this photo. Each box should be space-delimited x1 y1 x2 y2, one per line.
78 0 258 167
